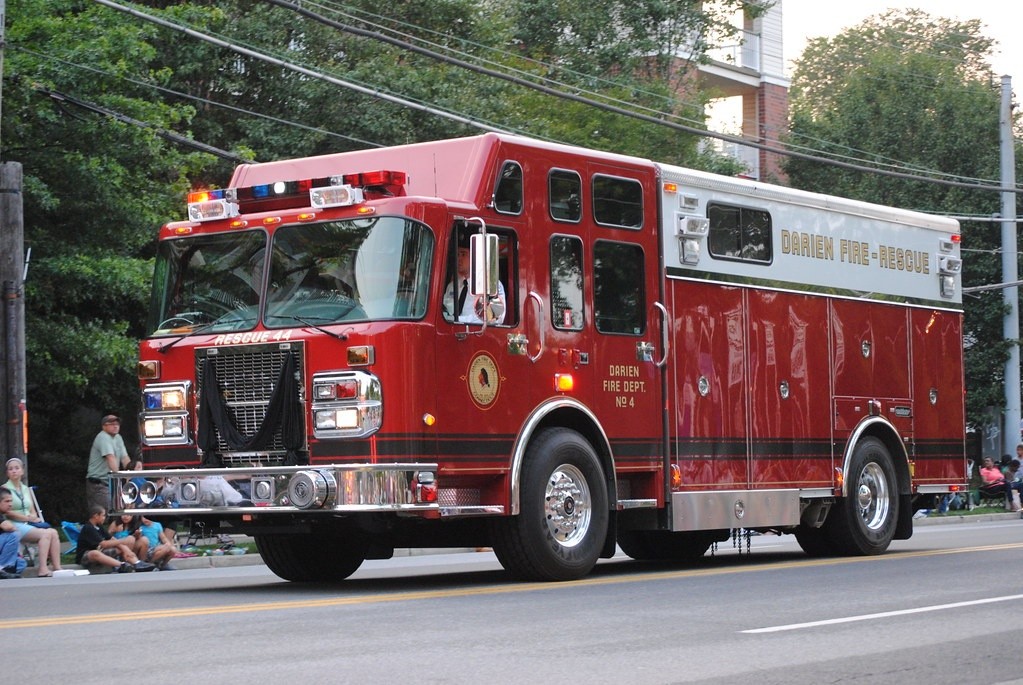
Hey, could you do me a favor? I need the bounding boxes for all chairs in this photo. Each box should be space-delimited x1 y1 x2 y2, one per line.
62 520 83 555
17 486 46 567
186 518 221 546
978 463 1006 508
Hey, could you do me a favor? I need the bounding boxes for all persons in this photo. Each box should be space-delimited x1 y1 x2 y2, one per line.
0 457 64 577
85 414 131 523
124 459 181 543
440 239 506 326
976 444 1022 515
76 505 156 574
135 515 178 571
109 514 149 570
0 487 27 579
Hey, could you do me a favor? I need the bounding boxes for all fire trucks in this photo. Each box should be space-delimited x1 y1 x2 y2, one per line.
112 131 971 587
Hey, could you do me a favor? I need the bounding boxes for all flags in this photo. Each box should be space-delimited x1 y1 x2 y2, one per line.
669 282 845 417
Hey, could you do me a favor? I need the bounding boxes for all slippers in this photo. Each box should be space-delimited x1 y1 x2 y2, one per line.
38 570 54 577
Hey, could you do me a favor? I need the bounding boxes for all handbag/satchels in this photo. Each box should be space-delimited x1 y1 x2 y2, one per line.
28 521 52 529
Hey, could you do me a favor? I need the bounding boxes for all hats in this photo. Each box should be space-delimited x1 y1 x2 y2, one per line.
102 415 120 424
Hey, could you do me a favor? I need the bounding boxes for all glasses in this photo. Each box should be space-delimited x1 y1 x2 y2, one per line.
103 423 120 427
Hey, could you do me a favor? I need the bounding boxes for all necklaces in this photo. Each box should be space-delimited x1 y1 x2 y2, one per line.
13 489 25 512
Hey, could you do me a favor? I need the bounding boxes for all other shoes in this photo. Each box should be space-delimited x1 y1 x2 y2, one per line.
0 570 11 579
160 564 177 571
134 560 157 571
117 562 135 573
11 573 22 578
1010 505 1022 512
